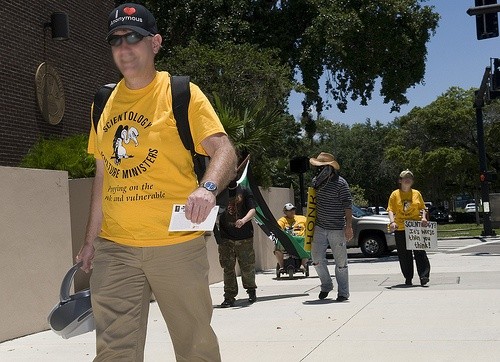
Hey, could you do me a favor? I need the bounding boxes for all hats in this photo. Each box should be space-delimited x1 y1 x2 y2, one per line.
310 152 340 170
282 203 296 210
104 3 157 41
399 170 414 178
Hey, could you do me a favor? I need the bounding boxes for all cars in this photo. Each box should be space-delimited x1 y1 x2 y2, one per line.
358 201 456 224
327 203 397 258
464 203 479 213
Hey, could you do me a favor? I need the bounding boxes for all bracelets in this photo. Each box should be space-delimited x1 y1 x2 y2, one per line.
389 221 394 226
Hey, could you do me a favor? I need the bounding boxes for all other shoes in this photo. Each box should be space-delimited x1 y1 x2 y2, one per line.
421 278 429 286
337 296 346 301
220 302 234 307
405 280 411 286
300 265 307 271
279 268 285 271
319 293 327 298
248 292 257 302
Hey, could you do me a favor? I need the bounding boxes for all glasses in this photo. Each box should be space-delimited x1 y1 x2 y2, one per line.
108 32 143 46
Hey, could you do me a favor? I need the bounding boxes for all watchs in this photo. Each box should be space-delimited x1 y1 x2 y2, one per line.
200 181 218 197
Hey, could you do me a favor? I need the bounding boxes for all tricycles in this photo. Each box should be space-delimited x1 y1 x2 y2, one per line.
275 236 310 280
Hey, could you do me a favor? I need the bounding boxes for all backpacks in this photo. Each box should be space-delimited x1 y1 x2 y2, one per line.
93 76 228 215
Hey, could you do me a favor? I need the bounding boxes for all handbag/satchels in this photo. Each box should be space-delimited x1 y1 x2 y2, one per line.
48 262 106 339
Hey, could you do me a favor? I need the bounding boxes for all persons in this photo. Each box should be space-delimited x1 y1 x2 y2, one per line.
77 3 238 362
309 152 354 302
274 203 308 270
213 177 257 307
387 171 430 285
